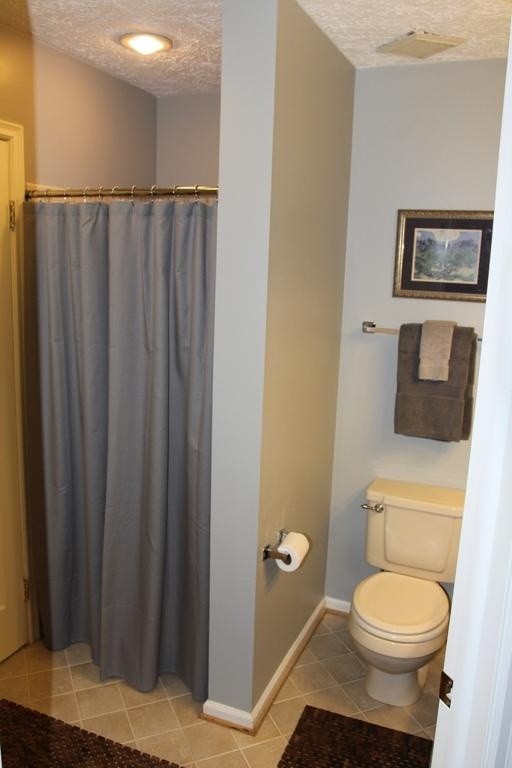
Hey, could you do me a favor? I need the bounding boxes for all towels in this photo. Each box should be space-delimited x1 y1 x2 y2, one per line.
393 323 477 443
417 320 458 383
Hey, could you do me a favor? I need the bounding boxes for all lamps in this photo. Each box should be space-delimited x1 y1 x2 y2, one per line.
119 33 172 56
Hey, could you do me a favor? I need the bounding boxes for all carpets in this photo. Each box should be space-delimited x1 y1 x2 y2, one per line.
276 705 434 768
0 699 190 768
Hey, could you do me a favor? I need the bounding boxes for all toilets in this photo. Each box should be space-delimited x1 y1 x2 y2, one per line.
347 477 466 706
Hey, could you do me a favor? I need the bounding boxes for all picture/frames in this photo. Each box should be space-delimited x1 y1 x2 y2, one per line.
391 209 494 303
